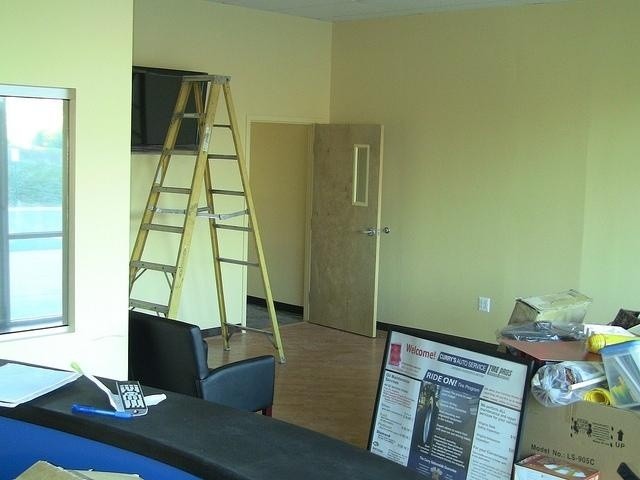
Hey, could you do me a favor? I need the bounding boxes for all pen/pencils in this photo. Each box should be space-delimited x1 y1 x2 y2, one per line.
72 405 135 419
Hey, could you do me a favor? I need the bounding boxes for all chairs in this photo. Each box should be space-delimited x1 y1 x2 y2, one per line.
130 309 275 420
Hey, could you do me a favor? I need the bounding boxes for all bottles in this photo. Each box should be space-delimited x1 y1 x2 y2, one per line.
585 333 639 355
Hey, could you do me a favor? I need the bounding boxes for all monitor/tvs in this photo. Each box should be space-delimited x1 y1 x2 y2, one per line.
132 64 210 154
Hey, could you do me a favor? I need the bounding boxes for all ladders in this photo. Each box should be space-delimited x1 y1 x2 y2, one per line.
129 75 286 364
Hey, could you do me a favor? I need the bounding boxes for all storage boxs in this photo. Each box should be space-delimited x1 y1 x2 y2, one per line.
514 384 640 480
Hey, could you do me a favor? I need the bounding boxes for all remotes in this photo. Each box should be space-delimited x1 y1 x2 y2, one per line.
115 380 148 417
